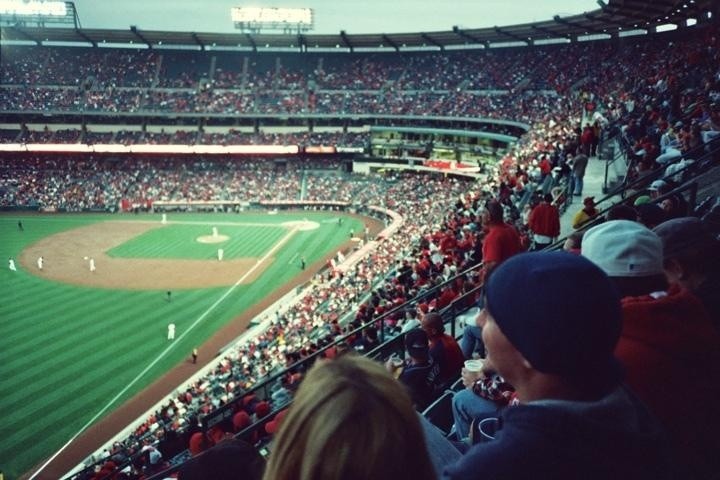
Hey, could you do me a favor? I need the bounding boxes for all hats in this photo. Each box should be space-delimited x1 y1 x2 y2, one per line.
189 394 290 454
648 179 666 191
651 216 711 262
403 332 429 359
486 250 623 378
581 219 665 279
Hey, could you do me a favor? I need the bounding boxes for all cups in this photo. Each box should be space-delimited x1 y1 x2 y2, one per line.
477 416 499 444
463 359 483 377
390 357 404 380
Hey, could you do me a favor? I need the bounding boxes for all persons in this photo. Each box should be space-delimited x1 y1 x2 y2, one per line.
1 36 720 480
